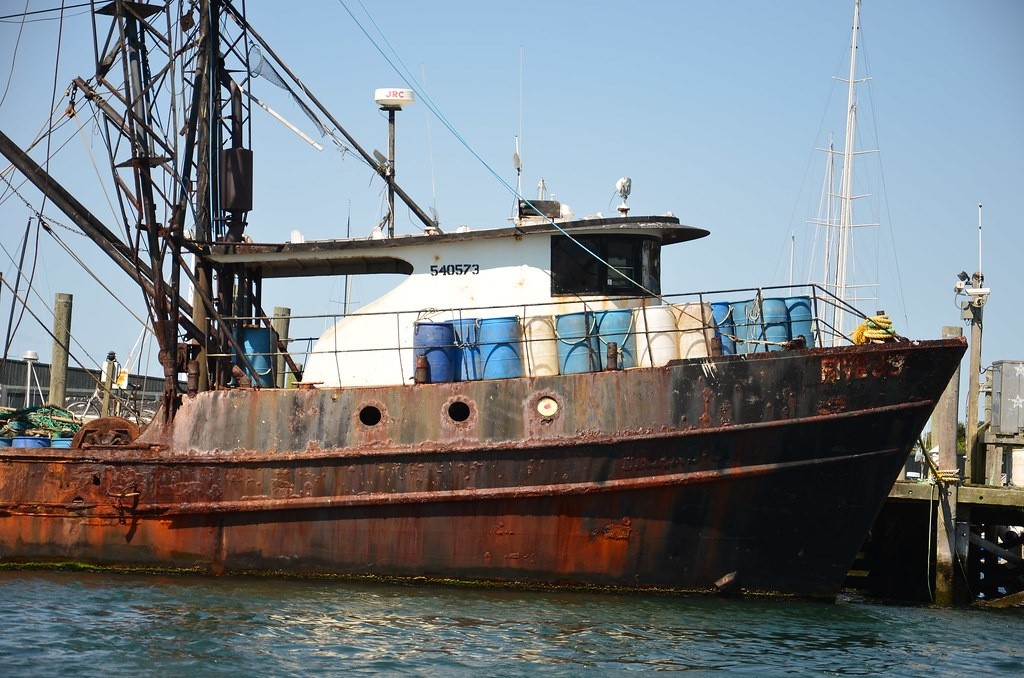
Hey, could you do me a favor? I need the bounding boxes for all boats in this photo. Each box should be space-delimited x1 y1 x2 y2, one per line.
0 0 973 606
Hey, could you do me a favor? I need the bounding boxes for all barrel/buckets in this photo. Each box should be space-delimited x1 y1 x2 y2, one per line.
595 308 636 372
0 433 73 451
445 319 483 381
784 294 816 350
728 300 749 355
478 315 523 380
231 324 279 388
520 316 559 377
635 305 680 368
412 322 454 383
671 302 720 359
555 311 602 374
747 298 789 355
710 301 736 355
